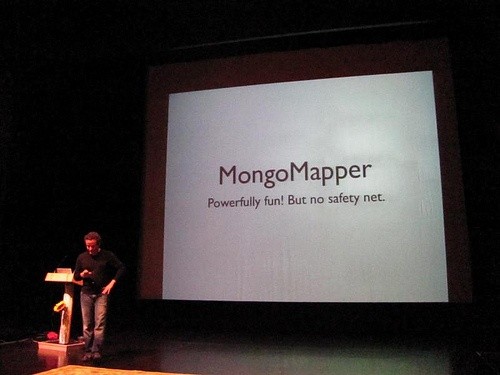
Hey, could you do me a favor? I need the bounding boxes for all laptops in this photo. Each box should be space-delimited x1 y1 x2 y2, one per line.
57 268 72 274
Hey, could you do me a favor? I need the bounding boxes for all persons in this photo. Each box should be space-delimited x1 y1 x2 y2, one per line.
74 231 125 361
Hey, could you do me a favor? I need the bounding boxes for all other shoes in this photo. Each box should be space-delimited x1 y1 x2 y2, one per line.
82 354 91 361
93 352 101 359
